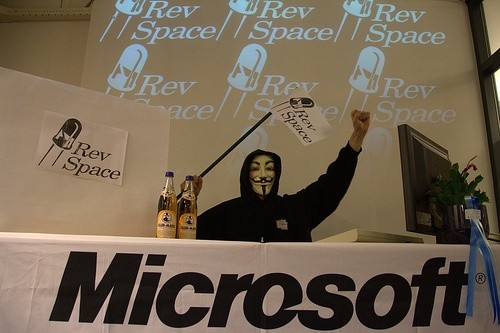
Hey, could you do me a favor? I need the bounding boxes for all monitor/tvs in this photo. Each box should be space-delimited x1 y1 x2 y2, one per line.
396 124 453 233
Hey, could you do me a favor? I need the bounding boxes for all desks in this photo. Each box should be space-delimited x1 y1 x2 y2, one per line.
2 229 499 332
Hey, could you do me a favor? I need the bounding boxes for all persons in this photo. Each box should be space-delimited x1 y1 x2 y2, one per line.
172 109 372 243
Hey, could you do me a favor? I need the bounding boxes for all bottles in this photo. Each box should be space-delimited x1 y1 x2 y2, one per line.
155 172 179 239
177 176 197 240
